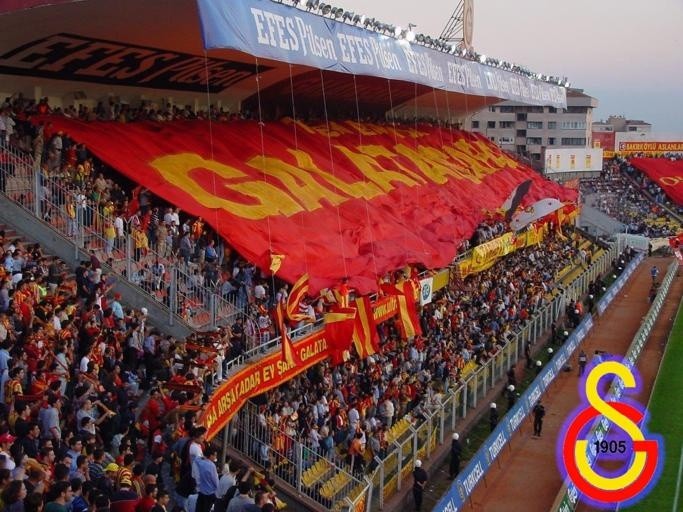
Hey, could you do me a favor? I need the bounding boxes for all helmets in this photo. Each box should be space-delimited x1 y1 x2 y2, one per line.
571 286 608 314
612 252 625 279
413 382 517 467
534 327 569 367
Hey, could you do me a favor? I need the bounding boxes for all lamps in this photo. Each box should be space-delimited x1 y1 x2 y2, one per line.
275 0 571 87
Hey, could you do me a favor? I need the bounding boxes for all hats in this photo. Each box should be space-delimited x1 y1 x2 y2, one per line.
0 433 17 443
103 463 120 472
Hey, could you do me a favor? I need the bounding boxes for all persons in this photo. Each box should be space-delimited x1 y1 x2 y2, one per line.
0 89 682 512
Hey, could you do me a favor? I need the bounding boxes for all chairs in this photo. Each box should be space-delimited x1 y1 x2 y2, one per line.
0 145 610 512
